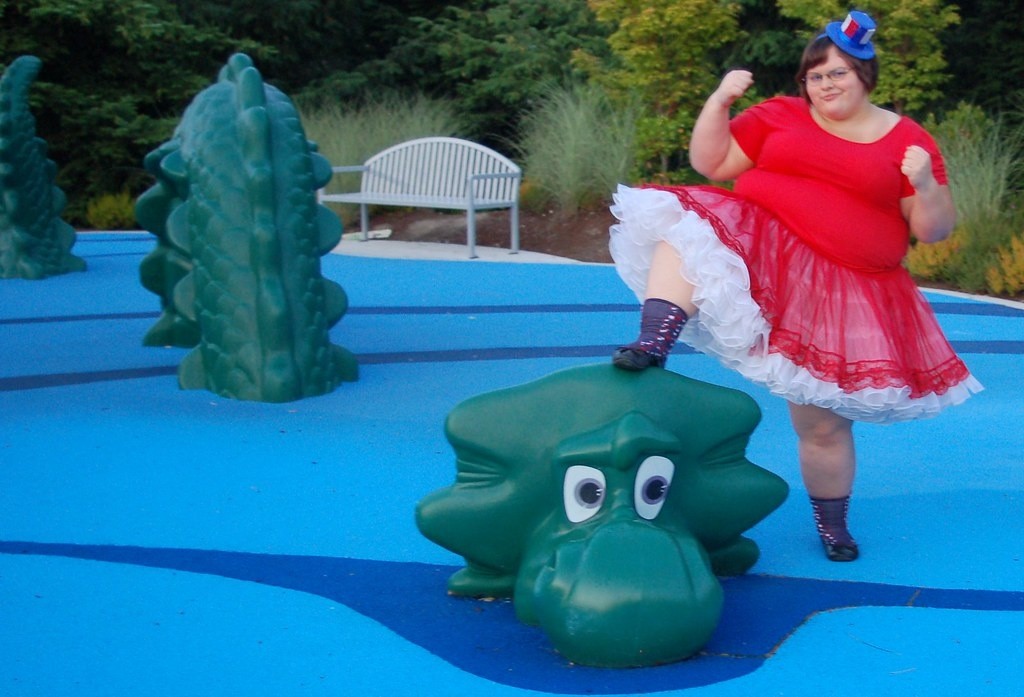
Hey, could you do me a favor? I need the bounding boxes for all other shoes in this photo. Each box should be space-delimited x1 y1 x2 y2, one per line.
612 346 667 371
818 533 860 563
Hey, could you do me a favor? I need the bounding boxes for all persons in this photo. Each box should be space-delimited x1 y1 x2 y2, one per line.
612 11 953 561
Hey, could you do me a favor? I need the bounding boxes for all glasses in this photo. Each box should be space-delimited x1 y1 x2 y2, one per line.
800 67 853 86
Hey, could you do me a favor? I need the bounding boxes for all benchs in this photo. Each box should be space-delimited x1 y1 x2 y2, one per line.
316 134 529 259
